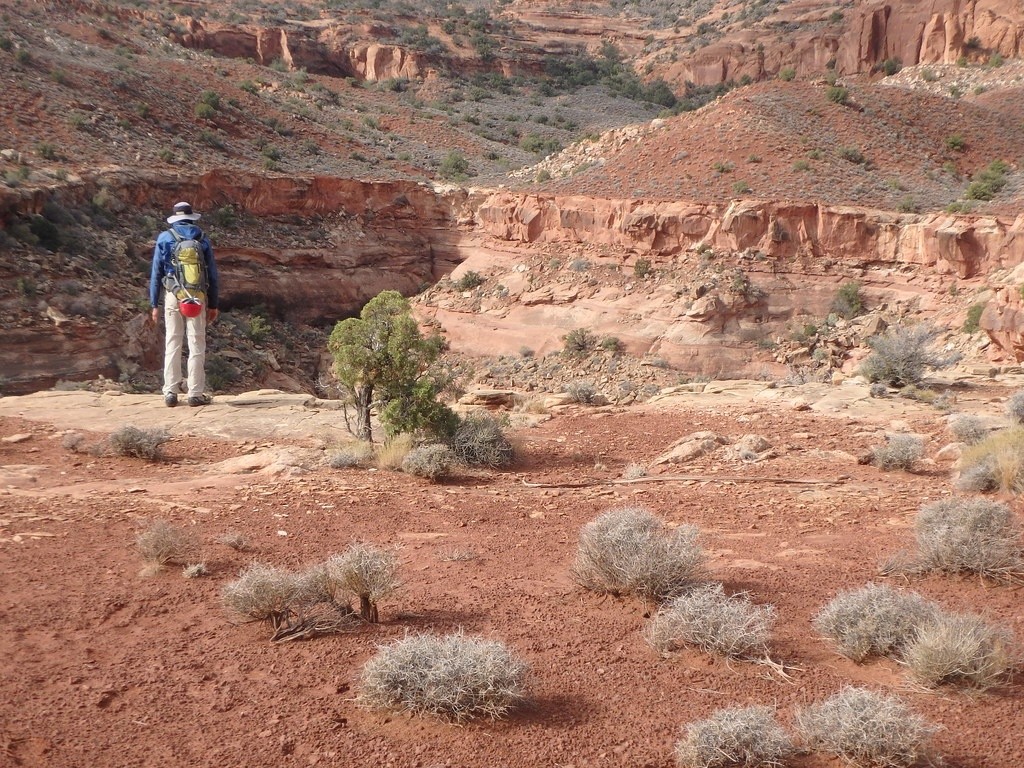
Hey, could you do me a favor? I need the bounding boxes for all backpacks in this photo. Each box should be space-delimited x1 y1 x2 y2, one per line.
168 228 206 302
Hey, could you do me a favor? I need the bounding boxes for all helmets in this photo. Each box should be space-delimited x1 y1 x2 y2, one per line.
177 300 204 317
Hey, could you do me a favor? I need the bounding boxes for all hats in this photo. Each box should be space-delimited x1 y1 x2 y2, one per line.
167 201 201 224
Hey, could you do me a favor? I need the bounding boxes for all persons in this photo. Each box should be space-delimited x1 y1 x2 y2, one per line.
149 201 219 407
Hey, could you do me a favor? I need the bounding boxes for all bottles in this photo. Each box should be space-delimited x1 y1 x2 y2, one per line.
166 268 175 292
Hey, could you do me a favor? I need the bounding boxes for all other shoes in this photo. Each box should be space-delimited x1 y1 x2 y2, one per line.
188 394 211 407
165 392 179 406
